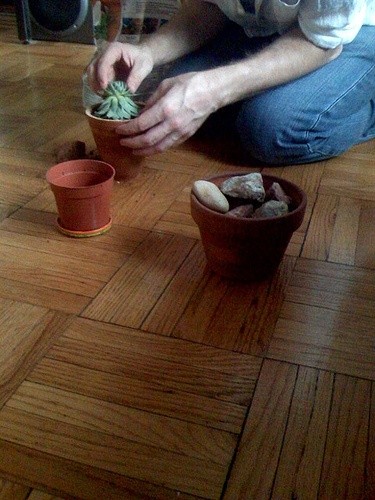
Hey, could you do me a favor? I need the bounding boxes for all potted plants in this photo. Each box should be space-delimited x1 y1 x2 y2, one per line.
85 80 154 182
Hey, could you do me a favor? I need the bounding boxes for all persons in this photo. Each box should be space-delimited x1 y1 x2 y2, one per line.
80 0 375 167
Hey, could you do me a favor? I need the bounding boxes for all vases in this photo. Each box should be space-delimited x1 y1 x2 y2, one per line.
190 172 308 289
46 160 114 240
